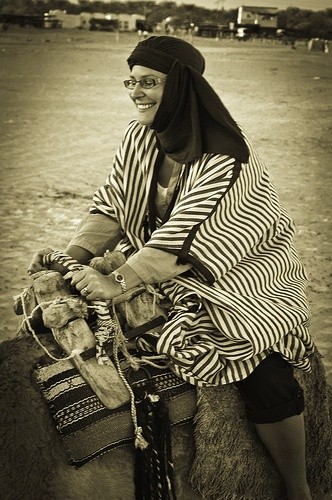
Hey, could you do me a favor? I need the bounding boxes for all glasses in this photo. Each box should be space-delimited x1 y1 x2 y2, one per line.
123 76 166 89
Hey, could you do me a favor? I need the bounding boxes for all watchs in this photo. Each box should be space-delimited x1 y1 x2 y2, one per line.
112 270 127 293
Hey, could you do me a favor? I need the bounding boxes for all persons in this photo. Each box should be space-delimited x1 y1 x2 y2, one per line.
27 37 313 500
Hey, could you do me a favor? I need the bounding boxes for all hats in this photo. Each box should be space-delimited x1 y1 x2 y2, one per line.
127 36 205 76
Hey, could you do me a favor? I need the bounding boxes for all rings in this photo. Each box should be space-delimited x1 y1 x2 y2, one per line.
83 288 89 295
